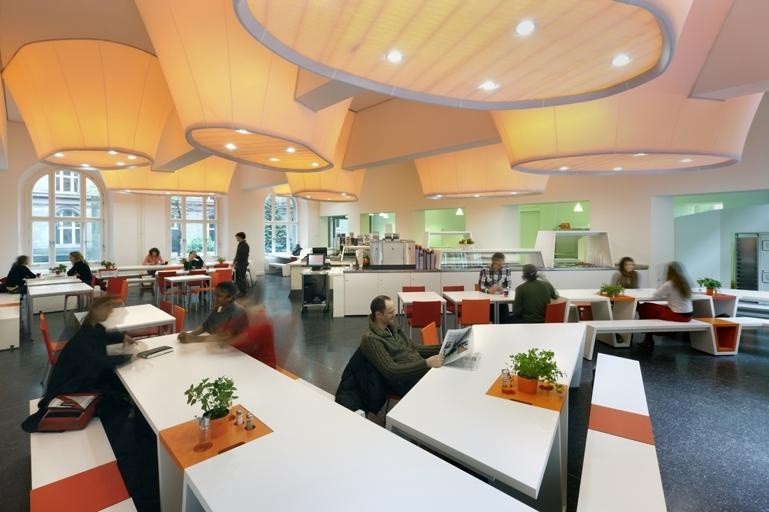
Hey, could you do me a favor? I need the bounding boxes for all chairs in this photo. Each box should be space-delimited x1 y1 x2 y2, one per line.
188 269 208 314
105 277 127 297
131 301 184 344
458 298 490 329
408 300 446 341
156 270 188 308
77 273 96 310
39 312 69 385
357 345 404 422
401 286 425 327
544 301 566 323
211 269 232 301
112 280 127 305
442 284 465 313
421 322 441 345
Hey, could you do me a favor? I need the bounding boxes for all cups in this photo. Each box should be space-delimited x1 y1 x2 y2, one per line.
501 369 511 390
198 417 211 444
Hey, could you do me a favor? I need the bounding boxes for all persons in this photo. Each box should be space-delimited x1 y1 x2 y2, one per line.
179 282 248 344
144 248 166 276
184 252 203 270
640 262 693 321
45 296 136 446
5 256 36 294
611 257 639 289
480 253 512 293
359 295 446 395
233 231 249 297
67 252 91 285
415 245 435 270
503 265 559 324
221 284 277 369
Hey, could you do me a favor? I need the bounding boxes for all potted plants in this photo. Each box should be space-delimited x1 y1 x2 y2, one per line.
216 256 225 265
696 277 723 296
595 280 624 298
48 263 68 276
458 238 474 250
508 347 567 395
179 256 189 266
101 260 116 274
184 375 239 439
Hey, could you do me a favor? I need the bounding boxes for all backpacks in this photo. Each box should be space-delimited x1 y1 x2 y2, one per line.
36 388 104 432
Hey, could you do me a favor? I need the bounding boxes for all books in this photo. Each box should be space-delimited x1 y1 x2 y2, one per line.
138 346 172 359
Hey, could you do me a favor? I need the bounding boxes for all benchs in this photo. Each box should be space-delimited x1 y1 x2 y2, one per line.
577 316 768 361
28 396 138 512
268 262 288 277
0 290 21 352
575 352 668 512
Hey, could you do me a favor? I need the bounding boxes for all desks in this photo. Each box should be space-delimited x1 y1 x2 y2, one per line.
289 261 353 290
328 264 649 318
89 262 254 297
442 290 516 331
25 273 93 340
397 290 447 334
551 286 769 348
276 252 303 262
386 323 588 512
73 304 177 339
105 330 541 512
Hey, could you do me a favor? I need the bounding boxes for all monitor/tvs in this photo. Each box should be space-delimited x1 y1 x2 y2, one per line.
312 247 327 259
307 253 325 271
